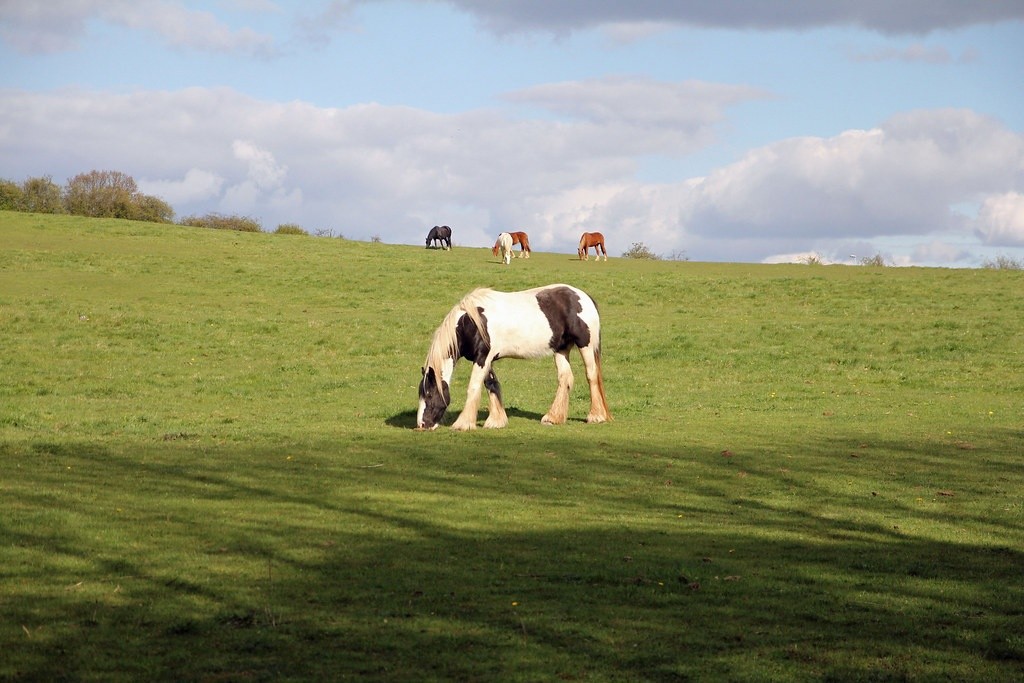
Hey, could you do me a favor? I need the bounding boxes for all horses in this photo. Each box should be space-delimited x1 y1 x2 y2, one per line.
412 283 617 432
425 226 452 252
577 232 608 262
491 231 531 264
